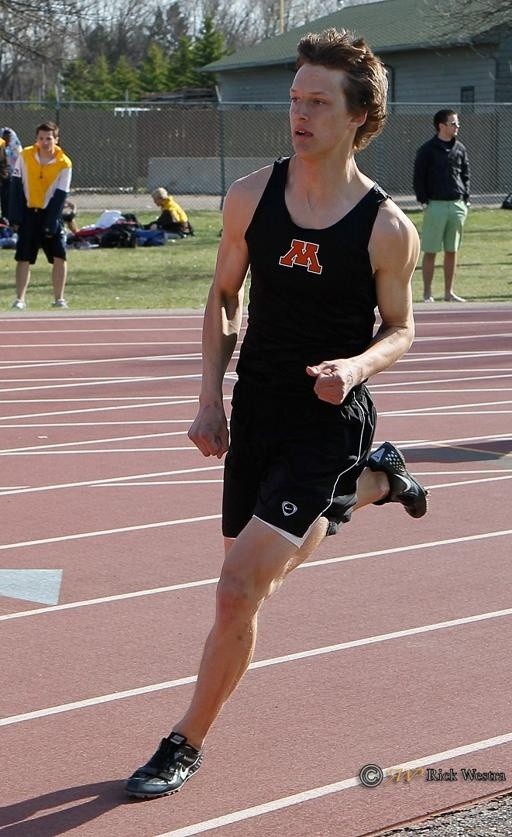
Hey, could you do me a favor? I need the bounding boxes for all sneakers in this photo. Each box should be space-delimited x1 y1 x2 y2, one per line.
11 299 26 310
124 731 204 798
367 439 427 518
55 299 69 309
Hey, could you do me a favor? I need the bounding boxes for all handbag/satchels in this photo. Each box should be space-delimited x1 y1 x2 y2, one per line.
63 209 184 248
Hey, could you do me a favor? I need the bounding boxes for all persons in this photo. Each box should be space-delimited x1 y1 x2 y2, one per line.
0 128 16 183
412 108 472 305
0 186 197 248
10 120 74 311
119 25 431 802
